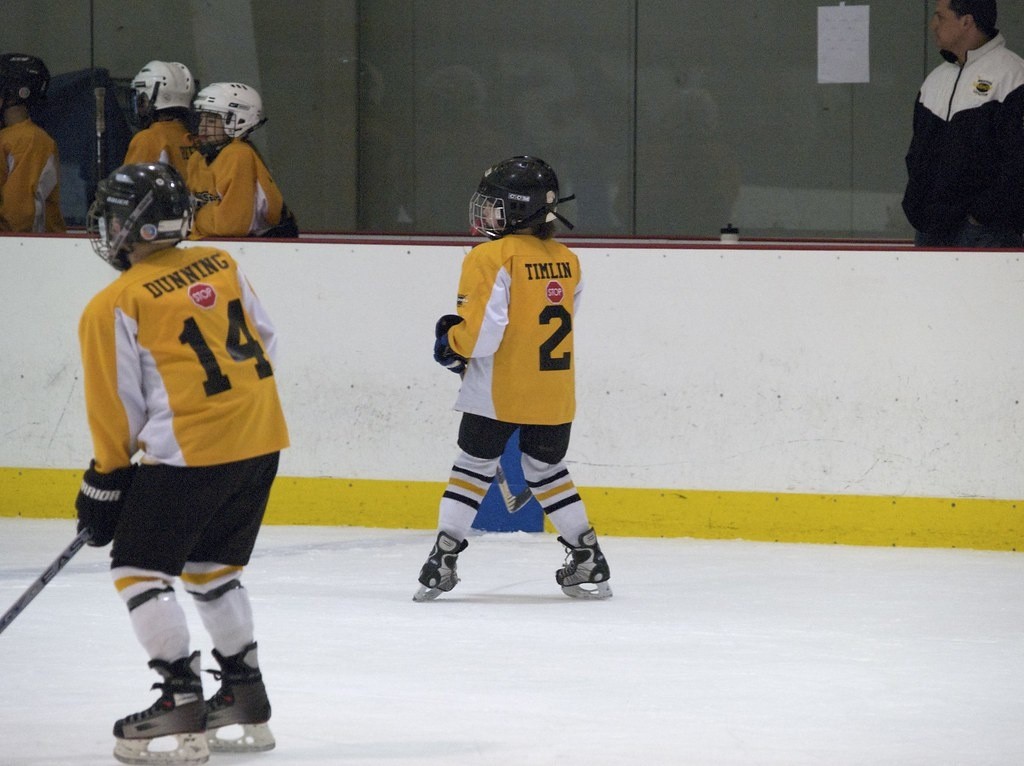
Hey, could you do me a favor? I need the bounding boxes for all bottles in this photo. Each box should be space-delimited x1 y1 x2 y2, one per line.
719 223 740 243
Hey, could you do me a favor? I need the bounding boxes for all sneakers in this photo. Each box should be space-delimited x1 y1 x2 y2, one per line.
112 651 210 766
555 526 613 600
206 641 275 754
412 530 469 602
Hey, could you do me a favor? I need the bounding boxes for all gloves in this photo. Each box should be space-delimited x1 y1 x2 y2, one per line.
433 335 467 374
76 460 131 546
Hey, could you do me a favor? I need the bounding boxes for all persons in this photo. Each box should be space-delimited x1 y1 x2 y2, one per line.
902 0 1024 247
185 82 301 238
0 52 67 234
123 60 197 183
412 155 613 601
75 161 290 766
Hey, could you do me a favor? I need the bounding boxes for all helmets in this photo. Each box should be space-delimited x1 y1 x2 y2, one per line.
0 53 50 106
191 82 267 156
86 160 194 271
468 154 560 240
128 61 195 127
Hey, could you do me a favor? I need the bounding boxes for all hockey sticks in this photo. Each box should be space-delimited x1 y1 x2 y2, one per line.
459 364 532 515
0 523 94 633
93 86 108 182
